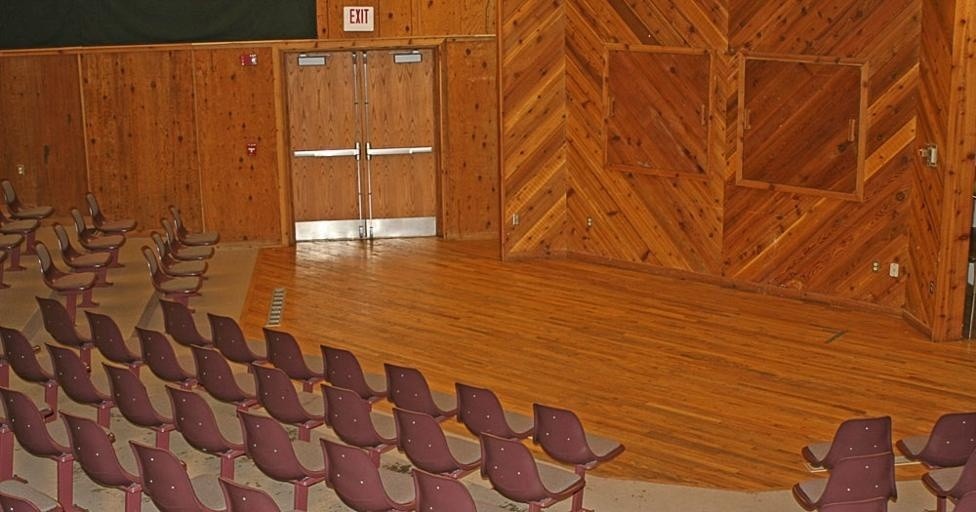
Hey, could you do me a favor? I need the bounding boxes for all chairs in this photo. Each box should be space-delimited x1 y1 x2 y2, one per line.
790 411 976 512
0 176 219 512
217 310 628 512
